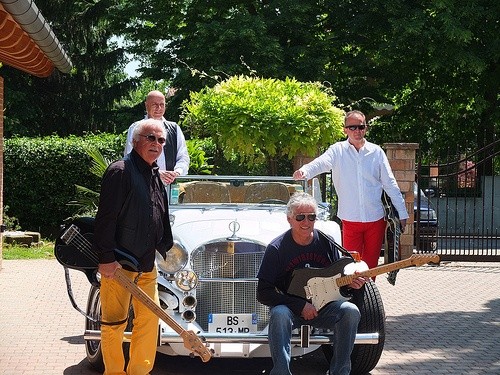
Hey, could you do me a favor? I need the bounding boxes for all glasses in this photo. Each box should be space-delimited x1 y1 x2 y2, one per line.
344 124 367 132
138 131 167 145
291 211 317 223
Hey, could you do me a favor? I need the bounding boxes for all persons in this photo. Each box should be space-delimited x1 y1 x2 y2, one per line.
256 193 371 375
293 110 410 283
94 119 174 375
124 90 190 185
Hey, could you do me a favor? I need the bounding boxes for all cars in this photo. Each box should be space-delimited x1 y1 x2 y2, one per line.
83 174 387 375
414 181 440 251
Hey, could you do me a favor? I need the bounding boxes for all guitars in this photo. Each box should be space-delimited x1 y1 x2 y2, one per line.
280 251 439 317
381 189 406 285
54 216 215 362
